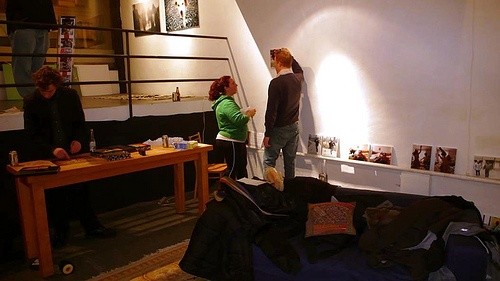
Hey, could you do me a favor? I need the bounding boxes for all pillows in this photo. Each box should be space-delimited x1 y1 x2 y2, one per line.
303 200 357 239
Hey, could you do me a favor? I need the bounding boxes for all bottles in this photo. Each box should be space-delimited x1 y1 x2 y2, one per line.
89 128 96 153
176 87 180 101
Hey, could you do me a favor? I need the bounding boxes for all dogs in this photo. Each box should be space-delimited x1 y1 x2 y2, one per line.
174 0 189 27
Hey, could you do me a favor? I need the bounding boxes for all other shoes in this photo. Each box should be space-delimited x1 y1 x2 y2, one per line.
52 222 72 249
84 225 116 239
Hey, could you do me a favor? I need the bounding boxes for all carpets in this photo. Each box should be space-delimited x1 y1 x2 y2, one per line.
85 239 211 281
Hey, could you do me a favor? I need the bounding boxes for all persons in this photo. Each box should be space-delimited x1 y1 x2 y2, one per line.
437 153 455 172
23 66 119 247
379 152 388 165
0 0 59 114
261 47 304 180
313 138 320 152
473 159 493 178
328 141 336 156
411 145 431 169
209 76 257 190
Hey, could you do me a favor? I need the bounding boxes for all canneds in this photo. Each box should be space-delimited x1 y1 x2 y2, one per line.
162 135 168 147
172 92 177 101
8 151 18 167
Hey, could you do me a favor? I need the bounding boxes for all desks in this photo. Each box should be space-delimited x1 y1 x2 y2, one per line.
6 140 214 281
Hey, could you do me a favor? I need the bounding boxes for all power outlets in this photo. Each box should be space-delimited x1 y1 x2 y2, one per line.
489 216 500 230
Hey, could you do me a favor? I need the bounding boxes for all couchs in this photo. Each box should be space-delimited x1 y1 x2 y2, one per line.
179 175 488 281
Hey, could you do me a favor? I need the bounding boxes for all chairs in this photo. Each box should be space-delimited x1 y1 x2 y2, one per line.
189 132 228 206
